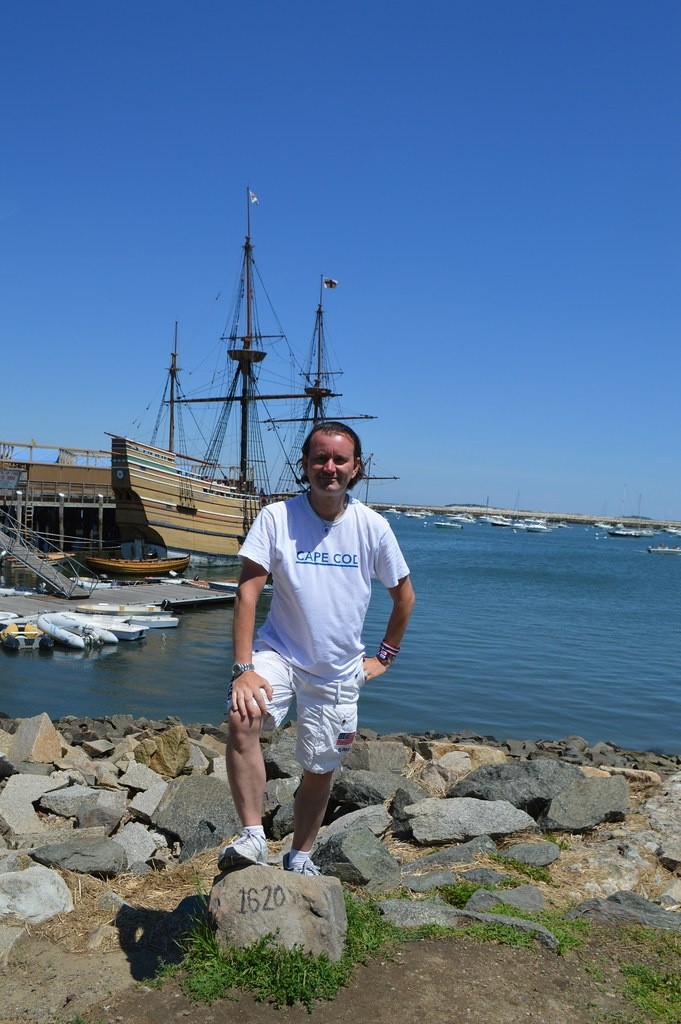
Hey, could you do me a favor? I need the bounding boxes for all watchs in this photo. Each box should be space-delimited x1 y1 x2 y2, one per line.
231 662 254 677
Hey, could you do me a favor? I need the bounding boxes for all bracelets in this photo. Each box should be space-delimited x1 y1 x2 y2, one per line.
376 638 401 665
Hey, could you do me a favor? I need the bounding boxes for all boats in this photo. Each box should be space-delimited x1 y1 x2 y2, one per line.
36 612 118 650
208 581 274 593
80 551 192 579
98 188 399 570
75 603 174 616
83 617 150 642
1 623 55 652
141 575 237 589
119 615 180 629
382 503 681 556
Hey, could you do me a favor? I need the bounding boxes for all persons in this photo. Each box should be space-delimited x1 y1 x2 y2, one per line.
203 476 218 494
217 422 415 877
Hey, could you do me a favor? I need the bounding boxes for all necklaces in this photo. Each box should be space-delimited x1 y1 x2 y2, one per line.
309 495 346 534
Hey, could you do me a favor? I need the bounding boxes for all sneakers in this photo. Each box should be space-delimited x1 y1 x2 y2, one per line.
217 827 268 872
282 852 321 876
